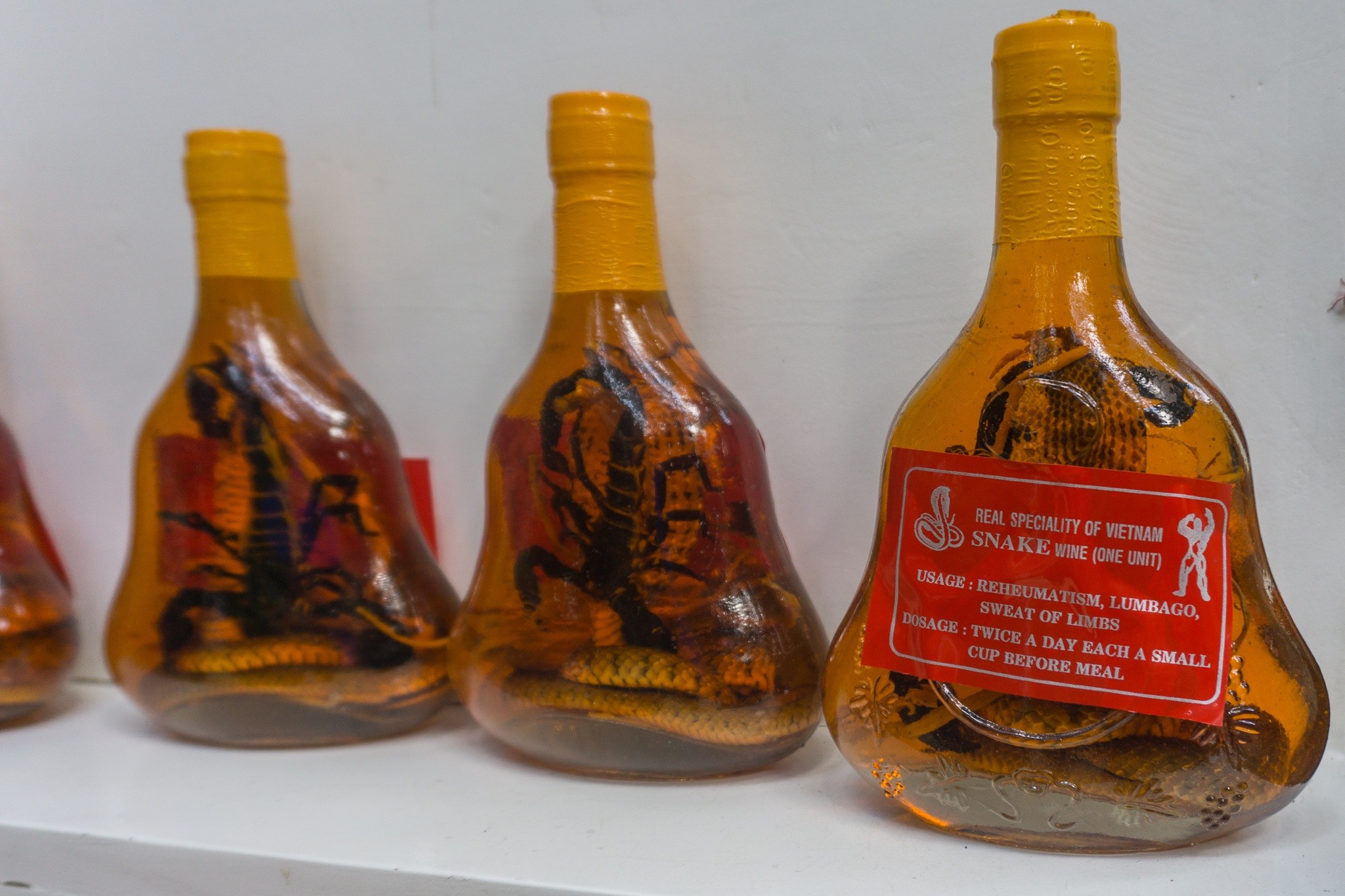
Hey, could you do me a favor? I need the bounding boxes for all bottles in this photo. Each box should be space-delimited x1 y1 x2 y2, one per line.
448 93 828 782
102 126 467 747
824 11 1332 857
0 417 89 719
